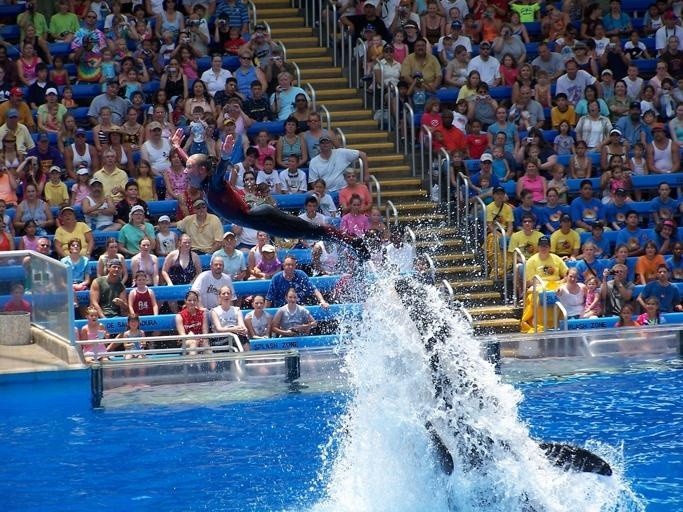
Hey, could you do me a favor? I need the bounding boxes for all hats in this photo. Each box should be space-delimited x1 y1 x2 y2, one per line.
615 188 624 195
539 214 570 243
363 1 375 31
38 133 48 142
319 136 332 143
662 11 676 20
7 87 58 117
262 244 275 253
610 102 664 134
76 121 161 136
131 200 205 223
452 21 461 29
480 153 504 193
224 119 235 125
223 231 234 239
50 166 103 185
572 43 613 75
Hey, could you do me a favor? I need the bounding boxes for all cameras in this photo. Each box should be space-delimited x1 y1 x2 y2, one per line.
256 32 264 37
183 38 191 43
168 67 177 72
480 96 486 100
663 90 669 94
608 43 616 49
279 87 288 92
610 269 618 275
527 138 533 142
273 57 281 61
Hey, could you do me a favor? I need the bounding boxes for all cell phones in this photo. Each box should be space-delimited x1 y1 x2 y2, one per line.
32 157 37 167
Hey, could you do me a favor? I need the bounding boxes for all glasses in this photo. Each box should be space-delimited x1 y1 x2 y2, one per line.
5 140 16 143
40 244 49 247
242 56 251 60
87 16 96 19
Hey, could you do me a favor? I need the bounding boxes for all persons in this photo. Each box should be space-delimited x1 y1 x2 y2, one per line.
424 0 683 359
2 1 445 389
